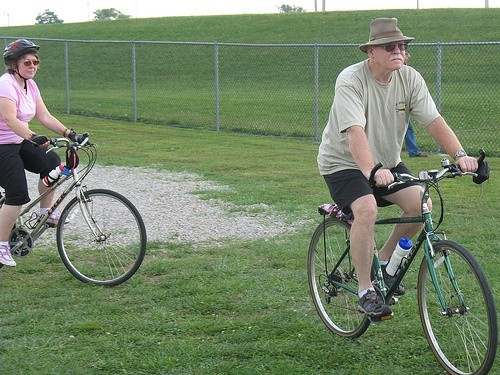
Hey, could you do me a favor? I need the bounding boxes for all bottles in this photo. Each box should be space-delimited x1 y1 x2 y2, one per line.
42 165 65 187
385 237 414 277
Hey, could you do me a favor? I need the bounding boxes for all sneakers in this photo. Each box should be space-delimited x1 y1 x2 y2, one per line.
372 263 405 296
36 209 71 224
0 241 16 266
357 289 394 321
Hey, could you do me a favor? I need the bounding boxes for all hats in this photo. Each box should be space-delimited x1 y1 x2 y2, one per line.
358 17 414 54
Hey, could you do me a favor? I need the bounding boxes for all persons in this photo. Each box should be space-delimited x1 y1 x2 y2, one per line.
405 120 427 156
0 38 89 266
316 17 478 317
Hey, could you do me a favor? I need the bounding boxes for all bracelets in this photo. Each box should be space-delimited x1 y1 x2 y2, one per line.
63 128 69 137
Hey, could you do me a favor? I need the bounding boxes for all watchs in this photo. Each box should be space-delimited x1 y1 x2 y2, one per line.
454 149 466 159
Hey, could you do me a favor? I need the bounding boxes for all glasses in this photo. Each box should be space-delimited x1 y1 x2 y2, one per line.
377 43 407 52
17 60 40 66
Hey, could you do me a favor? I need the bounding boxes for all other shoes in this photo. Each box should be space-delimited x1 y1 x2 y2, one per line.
416 152 430 157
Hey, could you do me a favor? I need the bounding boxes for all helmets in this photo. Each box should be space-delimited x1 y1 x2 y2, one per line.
1 38 41 59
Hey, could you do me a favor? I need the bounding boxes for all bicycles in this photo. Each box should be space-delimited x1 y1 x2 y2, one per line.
0 132 148 287
306 147 498 375
